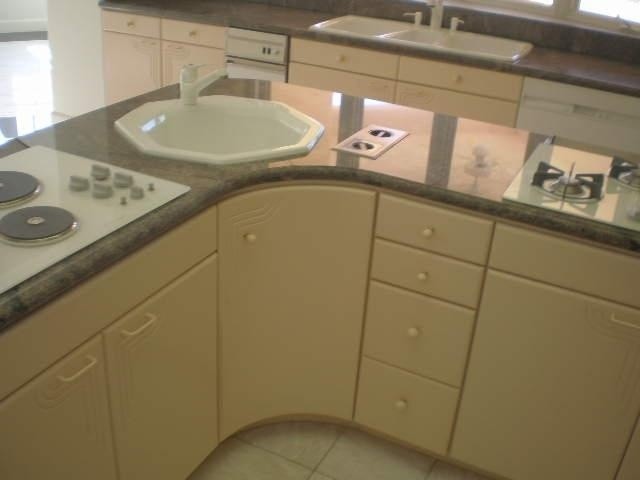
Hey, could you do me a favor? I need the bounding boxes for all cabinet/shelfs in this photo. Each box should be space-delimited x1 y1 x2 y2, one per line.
2 206 218 479
214 179 375 480
354 190 494 458
443 212 639 479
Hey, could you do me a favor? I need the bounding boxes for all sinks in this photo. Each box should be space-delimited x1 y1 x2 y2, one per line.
115 95 324 165
310 16 413 44
386 27 535 65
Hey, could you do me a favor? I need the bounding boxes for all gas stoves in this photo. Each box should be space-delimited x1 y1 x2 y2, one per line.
0 143 192 294
503 134 640 234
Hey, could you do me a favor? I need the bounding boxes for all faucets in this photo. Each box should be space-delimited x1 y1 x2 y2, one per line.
180 63 228 103
426 1 443 26
